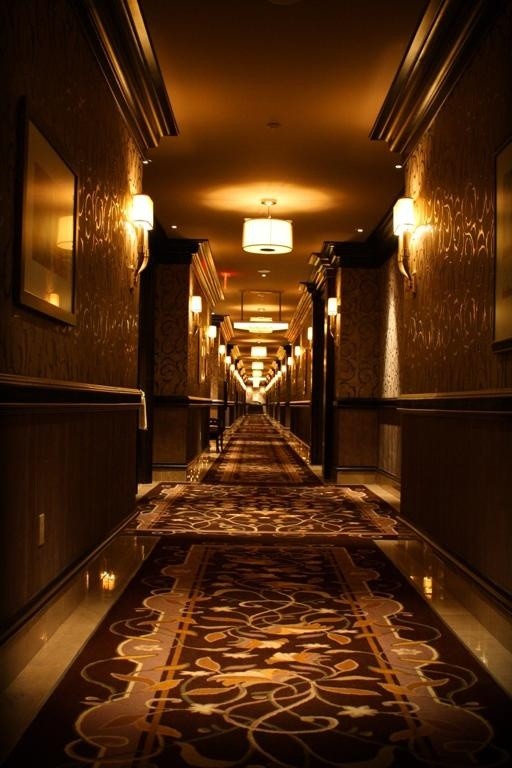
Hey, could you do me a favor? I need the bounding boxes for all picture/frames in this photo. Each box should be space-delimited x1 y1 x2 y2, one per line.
14 113 82 327
490 140 512 348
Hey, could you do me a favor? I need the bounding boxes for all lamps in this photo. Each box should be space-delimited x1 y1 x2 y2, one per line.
133 184 419 255
